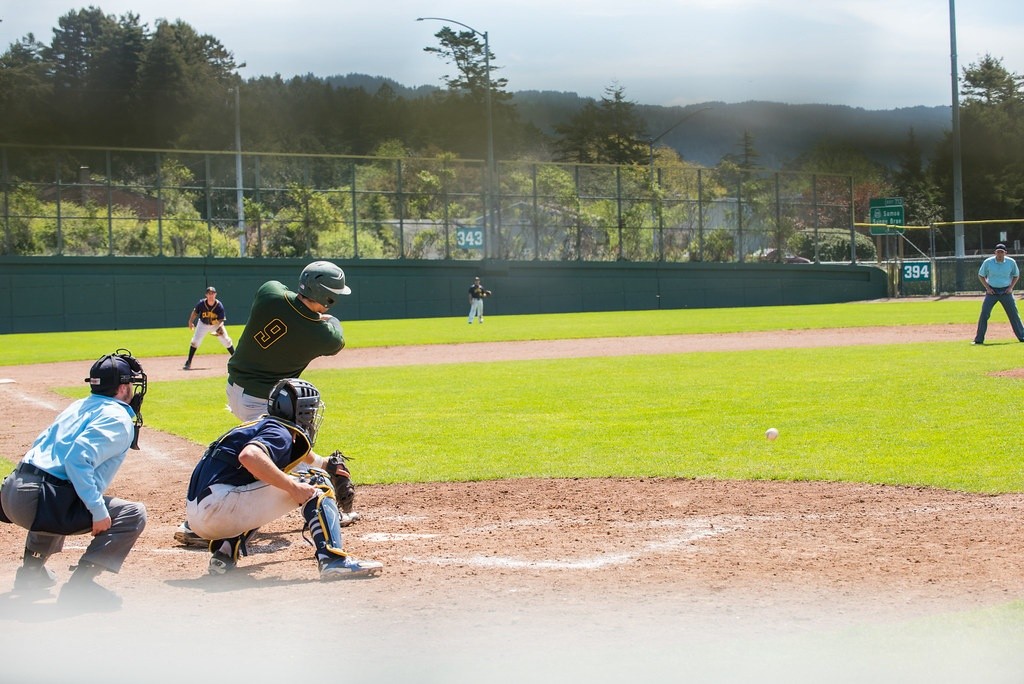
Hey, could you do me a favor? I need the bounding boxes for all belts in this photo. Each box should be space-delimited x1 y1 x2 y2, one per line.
197 488 212 505
15 463 64 487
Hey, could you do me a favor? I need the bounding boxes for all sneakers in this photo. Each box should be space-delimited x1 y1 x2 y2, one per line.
174 522 212 548
321 556 383 583
209 551 237 575
338 512 359 526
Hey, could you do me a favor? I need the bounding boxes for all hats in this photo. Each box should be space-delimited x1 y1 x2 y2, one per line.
90 356 133 385
994 244 1007 252
206 287 216 293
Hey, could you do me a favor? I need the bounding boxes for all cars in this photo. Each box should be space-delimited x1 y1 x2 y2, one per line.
749 249 810 264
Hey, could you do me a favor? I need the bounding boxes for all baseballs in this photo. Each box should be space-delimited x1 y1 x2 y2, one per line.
765 428 779 440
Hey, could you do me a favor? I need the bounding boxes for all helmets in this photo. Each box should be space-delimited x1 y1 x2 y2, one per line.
267 377 325 446
298 260 351 308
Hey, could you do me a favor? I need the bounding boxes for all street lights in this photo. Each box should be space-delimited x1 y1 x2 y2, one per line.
416 17 496 257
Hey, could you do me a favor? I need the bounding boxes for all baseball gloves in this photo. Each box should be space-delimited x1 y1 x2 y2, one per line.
486 290 491 296
325 452 355 514
210 324 224 336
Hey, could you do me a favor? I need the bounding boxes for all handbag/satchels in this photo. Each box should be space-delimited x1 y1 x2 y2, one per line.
29 485 88 533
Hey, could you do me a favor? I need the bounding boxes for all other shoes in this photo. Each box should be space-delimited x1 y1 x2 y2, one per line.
13 566 58 592
55 582 123 613
182 361 192 370
971 341 983 345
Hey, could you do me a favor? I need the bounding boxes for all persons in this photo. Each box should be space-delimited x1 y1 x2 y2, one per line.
173 261 360 547
0 348 163 609
970 244 1024 345
183 286 235 370
468 276 491 324
186 377 383 581
